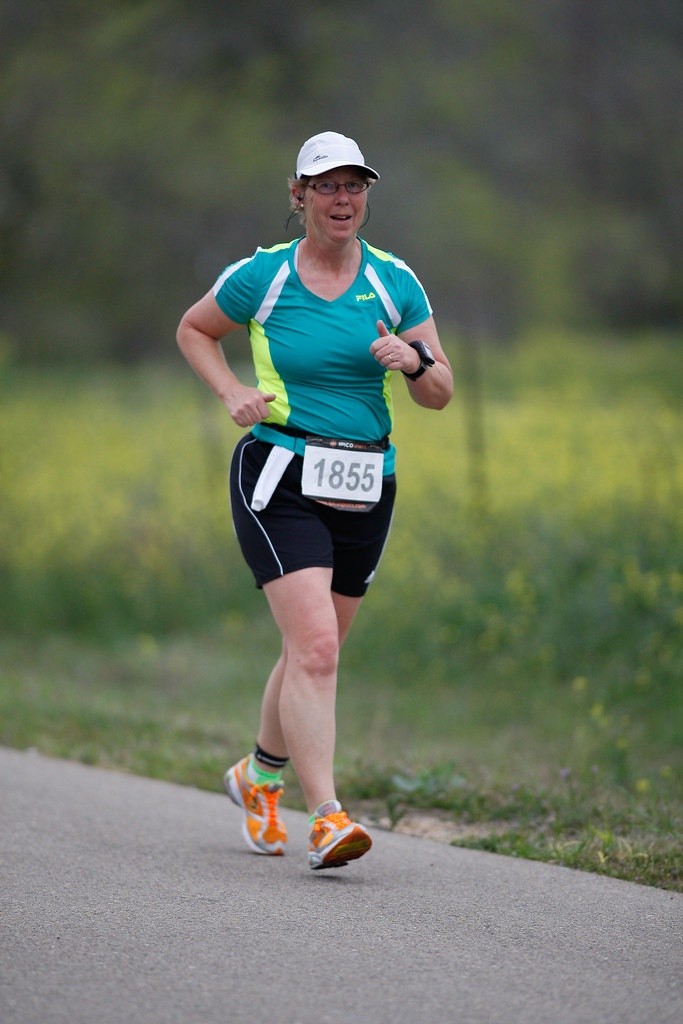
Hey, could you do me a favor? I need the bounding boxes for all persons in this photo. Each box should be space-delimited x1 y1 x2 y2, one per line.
176 130 459 871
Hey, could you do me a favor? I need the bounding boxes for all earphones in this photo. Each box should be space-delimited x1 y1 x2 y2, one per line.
297 194 303 201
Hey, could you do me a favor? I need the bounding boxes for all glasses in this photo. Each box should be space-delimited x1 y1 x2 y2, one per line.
301 182 370 193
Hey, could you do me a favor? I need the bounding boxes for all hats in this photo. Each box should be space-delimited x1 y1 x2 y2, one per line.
296 131 382 180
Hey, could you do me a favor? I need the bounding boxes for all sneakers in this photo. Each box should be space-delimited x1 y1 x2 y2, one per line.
223 754 288 855
307 799 373 870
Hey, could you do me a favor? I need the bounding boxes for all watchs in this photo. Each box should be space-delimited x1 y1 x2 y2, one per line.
400 339 434 382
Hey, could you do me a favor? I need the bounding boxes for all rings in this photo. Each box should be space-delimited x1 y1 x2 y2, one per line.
388 354 393 363
